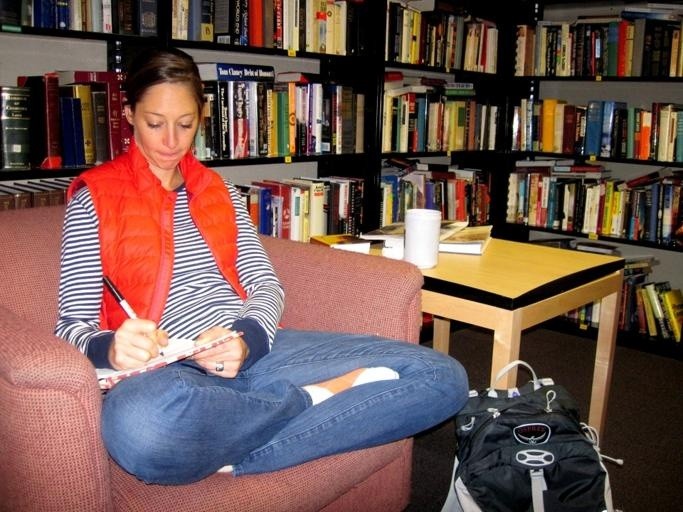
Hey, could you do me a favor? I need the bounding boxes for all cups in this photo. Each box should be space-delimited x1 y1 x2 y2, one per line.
404 208 442 270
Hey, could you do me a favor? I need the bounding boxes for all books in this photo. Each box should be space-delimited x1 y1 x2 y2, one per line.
0 1 154 214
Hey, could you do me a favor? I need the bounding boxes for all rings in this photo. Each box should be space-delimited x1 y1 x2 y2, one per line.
213 361 225 373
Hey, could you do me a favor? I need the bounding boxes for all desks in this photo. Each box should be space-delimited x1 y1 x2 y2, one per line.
366 234 627 447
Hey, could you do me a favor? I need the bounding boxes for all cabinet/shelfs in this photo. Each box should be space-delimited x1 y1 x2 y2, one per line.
0 0 683 360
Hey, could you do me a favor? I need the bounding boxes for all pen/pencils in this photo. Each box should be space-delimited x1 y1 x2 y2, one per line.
103 275 165 357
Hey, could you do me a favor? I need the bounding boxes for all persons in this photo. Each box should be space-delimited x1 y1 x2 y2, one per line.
53 36 470 487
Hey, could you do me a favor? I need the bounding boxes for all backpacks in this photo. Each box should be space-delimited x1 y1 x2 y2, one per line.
441 360 623 512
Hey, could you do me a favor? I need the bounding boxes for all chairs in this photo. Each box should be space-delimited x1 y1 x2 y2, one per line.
1 202 425 511
